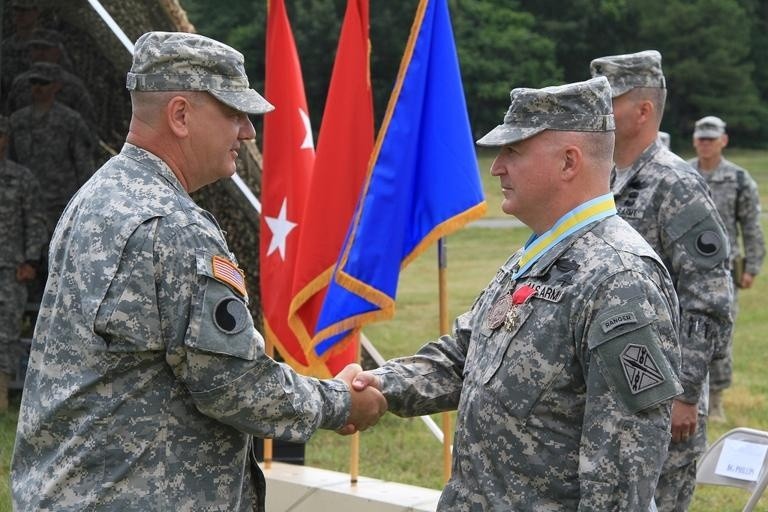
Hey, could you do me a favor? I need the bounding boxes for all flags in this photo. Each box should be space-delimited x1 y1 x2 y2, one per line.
259 3 488 384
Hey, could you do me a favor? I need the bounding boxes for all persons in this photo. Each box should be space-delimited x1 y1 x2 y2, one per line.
337 74 683 512
592 51 738 512
2 1 100 426
680 117 768 425
8 31 391 512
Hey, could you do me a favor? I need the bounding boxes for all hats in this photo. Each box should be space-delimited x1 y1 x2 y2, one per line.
588 49 667 100
124 31 275 115
0 0 64 135
692 115 727 140
475 73 617 147
